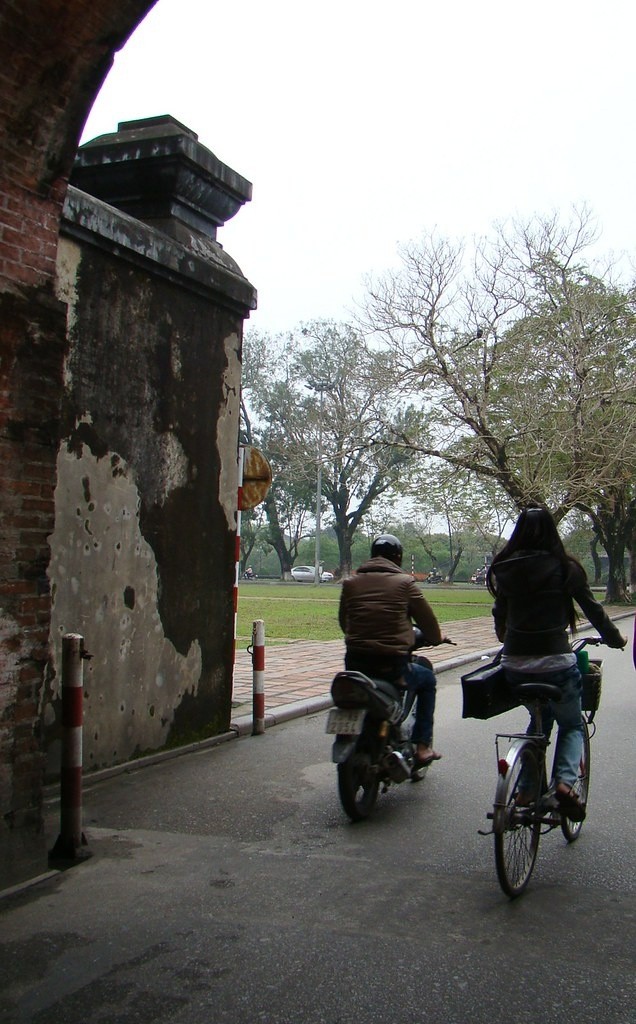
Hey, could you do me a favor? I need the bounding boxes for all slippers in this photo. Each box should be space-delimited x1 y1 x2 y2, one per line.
417 749 443 763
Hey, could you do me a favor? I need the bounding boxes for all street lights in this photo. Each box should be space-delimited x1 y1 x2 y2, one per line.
303 378 335 584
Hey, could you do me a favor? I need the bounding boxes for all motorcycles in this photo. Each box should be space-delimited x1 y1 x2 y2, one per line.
241 570 259 581
468 575 485 586
325 624 459 823
423 573 445 585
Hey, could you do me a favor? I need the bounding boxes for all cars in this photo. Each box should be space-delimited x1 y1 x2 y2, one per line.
290 565 335 583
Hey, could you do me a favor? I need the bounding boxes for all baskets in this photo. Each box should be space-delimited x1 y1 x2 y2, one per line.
572 657 603 712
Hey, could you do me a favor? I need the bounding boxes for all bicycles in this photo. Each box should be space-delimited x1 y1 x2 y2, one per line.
476 636 626 897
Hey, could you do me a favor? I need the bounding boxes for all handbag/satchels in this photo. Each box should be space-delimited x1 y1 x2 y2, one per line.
462 644 523 720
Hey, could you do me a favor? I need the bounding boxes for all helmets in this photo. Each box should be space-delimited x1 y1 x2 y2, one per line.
372 534 402 567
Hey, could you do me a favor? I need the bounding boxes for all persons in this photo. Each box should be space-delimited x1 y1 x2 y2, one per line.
427 567 436 581
246 565 253 577
491 503 628 821
337 535 450 765
476 568 482 582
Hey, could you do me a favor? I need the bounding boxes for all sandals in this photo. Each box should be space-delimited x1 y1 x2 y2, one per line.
560 783 587 822
